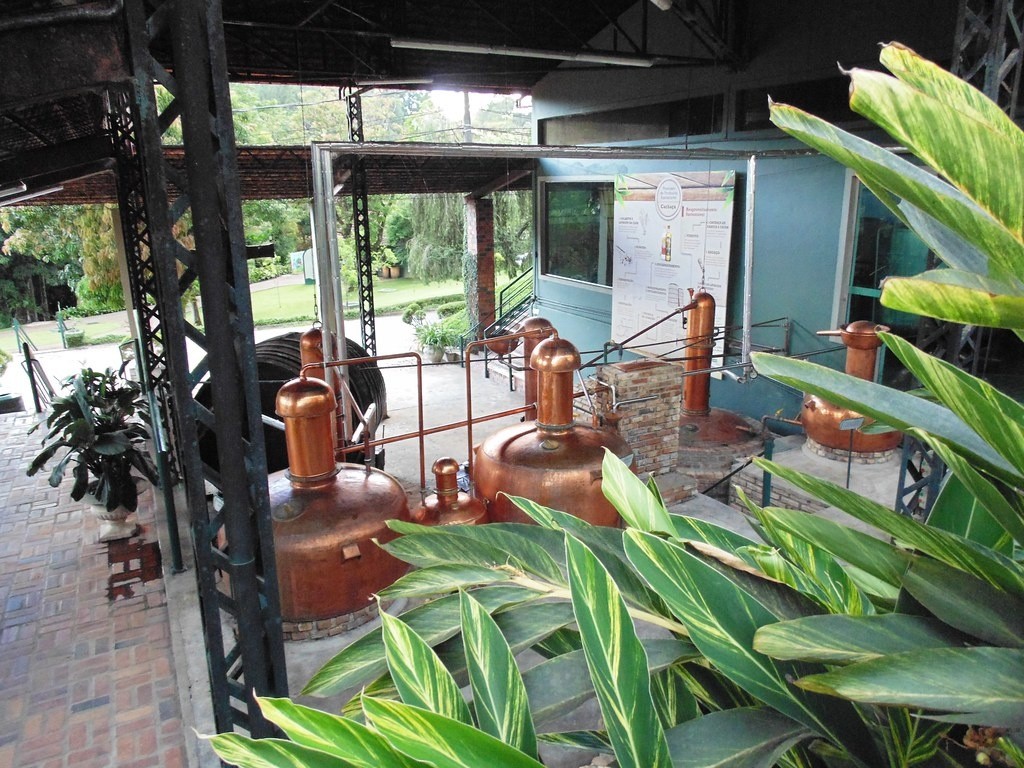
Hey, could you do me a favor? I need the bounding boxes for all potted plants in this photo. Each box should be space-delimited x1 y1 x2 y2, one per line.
26 360 160 542
409 323 451 363
372 248 402 278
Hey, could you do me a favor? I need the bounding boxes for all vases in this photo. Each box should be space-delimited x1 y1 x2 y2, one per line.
446 352 456 361
